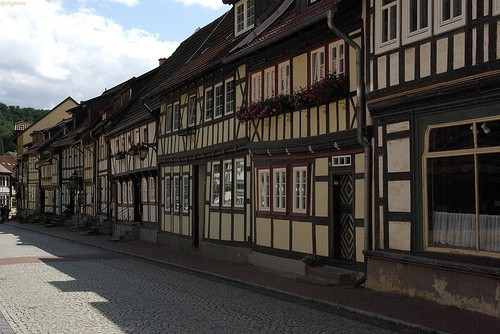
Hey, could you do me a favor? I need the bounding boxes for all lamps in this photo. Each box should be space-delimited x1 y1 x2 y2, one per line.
136 142 158 161
471 121 490 134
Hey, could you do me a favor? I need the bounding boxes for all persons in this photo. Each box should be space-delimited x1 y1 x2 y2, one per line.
0 203 12 222
